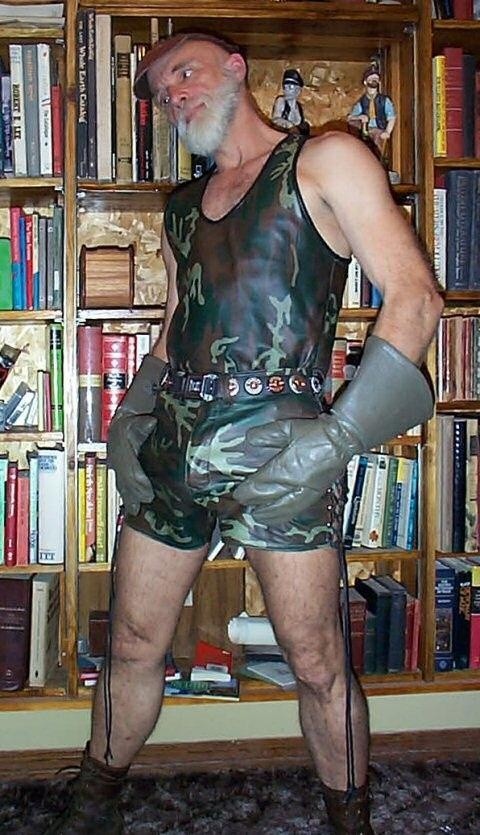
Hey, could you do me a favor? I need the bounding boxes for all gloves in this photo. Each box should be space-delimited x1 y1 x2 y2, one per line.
233 336 433 527
107 356 169 515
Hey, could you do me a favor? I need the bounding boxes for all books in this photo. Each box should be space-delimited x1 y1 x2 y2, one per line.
339 202 413 309
340 561 422 675
1 44 64 178
76 609 299 703
2 573 61 692
1 445 123 568
429 1 479 674
341 453 418 551
1 322 165 445
1 205 63 309
336 321 424 437
76 11 210 185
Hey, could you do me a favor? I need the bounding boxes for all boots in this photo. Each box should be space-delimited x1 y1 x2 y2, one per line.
320 781 374 835
43 741 130 834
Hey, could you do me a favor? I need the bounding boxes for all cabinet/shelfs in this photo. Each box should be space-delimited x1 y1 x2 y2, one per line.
76 4 429 699
431 18 480 683
0 0 75 703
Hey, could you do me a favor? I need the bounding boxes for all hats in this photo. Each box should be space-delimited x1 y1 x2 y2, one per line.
133 33 238 99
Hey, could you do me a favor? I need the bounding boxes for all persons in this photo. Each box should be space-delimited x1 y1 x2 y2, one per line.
37 28 448 835
272 69 310 138
347 64 400 185
